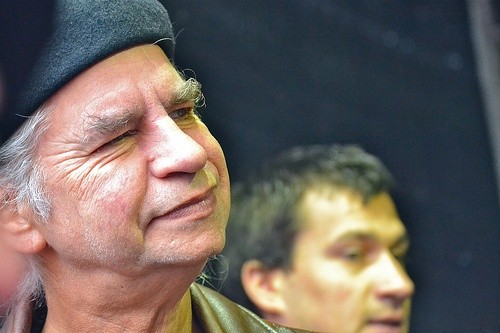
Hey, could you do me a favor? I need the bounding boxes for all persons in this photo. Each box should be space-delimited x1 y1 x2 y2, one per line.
218 145 414 333
0 1 312 333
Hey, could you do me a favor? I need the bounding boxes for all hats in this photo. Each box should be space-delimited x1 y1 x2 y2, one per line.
0 0 177 148
